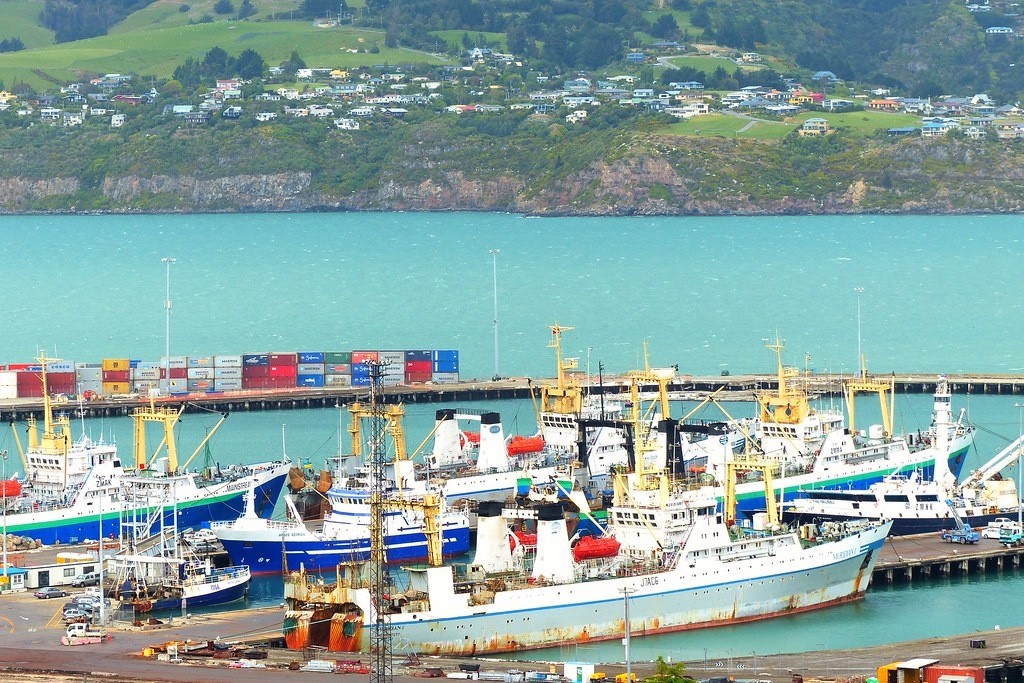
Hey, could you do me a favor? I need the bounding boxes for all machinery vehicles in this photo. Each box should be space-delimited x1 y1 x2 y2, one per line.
942 497 979 544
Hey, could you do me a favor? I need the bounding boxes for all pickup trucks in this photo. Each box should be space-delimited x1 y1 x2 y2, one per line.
987 517 1017 527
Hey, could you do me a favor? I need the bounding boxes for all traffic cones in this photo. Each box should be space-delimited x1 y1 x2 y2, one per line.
106 633 111 640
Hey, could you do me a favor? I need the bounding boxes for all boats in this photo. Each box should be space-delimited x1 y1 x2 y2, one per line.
171 391 189 397
574 538 620 560
0 481 22 497
508 435 546 455
1 323 1024 652
458 430 481 448
205 386 226 394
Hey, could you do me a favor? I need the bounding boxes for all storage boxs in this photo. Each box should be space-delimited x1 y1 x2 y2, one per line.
0 350 459 400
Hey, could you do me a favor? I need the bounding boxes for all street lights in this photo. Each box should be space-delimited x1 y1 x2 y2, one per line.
854 286 867 375
161 257 178 393
490 247 502 378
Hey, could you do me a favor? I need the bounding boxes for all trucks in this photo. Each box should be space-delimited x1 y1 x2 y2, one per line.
69 587 100 599
998 525 1024 547
66 622 108 637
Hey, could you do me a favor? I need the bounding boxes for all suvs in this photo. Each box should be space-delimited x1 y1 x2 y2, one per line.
61 596 107 621
71 573 100 587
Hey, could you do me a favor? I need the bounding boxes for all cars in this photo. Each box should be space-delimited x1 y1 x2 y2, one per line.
981 527 1000 540
34 586 69 599
